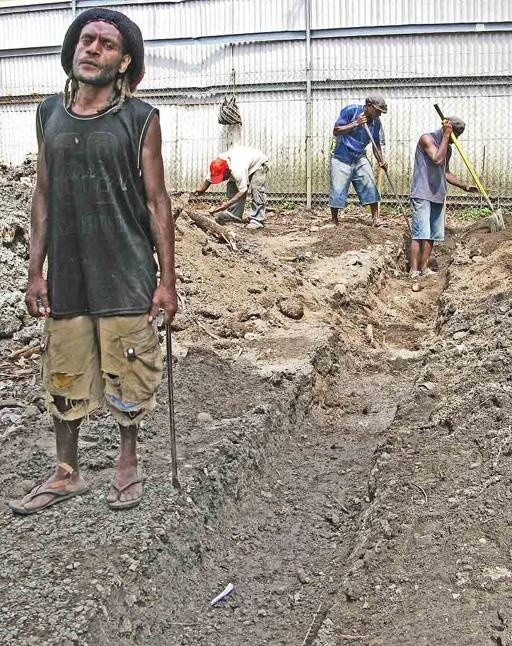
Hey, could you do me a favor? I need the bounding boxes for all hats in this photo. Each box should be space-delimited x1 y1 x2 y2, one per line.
444 115 465 136
211 160 227 183
365 94 388 115
60 6 145 93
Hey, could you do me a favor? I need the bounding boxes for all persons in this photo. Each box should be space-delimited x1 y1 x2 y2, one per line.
8 6 178 517
328 95 390 228
196 147 270 229
408 116 479 279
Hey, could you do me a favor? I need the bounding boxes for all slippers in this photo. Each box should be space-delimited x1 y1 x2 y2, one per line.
9 482 91 511
107 463 143 509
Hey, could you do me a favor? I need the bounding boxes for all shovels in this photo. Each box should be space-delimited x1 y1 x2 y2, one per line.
435 103 505 233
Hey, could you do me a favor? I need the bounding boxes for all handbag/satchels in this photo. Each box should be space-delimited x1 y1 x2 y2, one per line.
217 94 242 126
